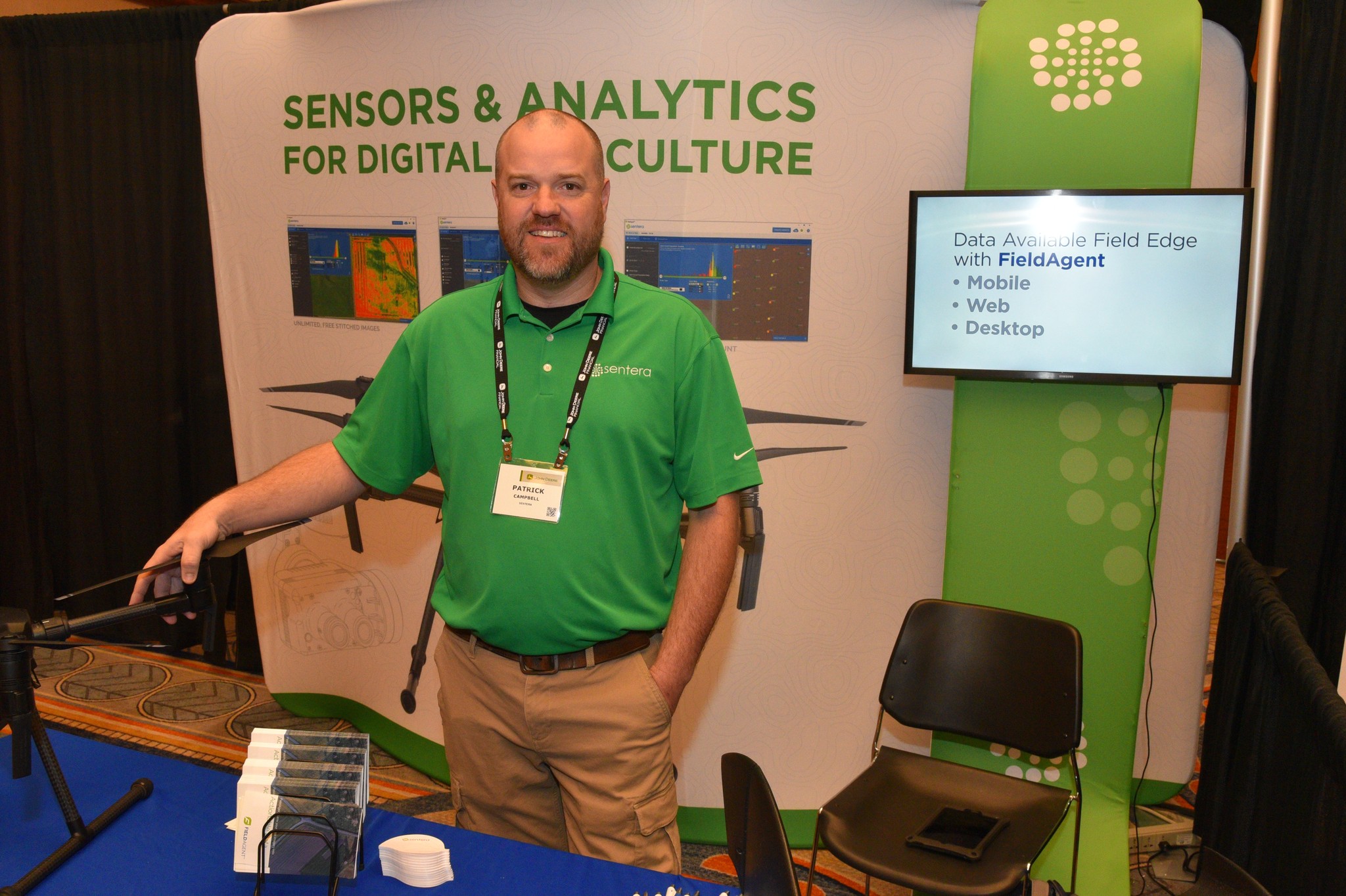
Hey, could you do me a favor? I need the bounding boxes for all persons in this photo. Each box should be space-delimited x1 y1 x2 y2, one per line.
128 108 763 874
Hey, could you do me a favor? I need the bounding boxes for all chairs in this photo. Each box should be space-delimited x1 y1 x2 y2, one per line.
799 597 1085 896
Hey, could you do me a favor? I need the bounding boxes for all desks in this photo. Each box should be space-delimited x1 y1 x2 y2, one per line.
3 720 746 896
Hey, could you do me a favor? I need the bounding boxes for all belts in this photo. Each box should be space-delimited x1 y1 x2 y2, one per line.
445 620 653 674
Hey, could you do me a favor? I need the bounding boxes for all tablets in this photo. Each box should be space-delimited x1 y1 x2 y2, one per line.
905 803 1009 862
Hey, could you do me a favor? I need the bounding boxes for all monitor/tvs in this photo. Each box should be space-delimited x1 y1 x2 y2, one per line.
905 188 1254 386
